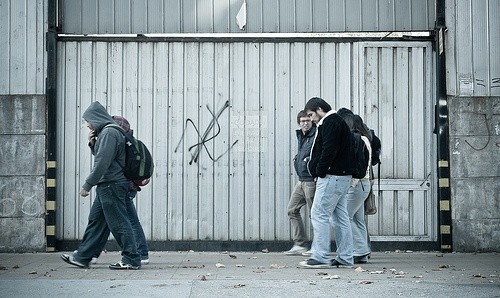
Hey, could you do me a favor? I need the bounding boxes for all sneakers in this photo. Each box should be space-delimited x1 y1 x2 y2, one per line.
60 253 90 268
109 260 141 270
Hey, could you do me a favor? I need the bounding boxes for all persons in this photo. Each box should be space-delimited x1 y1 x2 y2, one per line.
61 101 149 270
284 97 372 269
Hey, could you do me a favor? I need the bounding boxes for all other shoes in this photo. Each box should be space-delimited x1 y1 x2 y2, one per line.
141 258 150 264
299 258 329 269
74 250 98 264
284 244 312 256
329 259 353 269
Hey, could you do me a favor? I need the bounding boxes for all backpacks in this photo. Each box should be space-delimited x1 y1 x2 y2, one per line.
367 129 382 165
101 124 154 186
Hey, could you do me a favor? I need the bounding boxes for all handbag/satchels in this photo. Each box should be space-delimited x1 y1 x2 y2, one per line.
364 192 377 215
